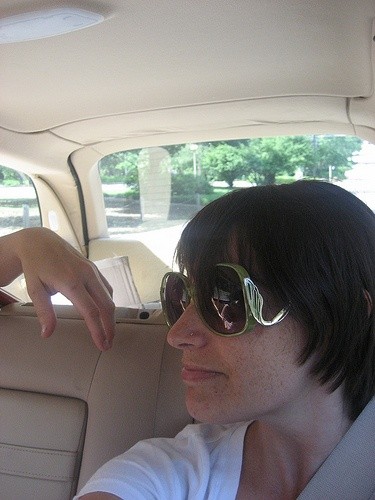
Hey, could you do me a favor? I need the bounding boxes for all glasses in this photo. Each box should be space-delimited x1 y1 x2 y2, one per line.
157 263 295 339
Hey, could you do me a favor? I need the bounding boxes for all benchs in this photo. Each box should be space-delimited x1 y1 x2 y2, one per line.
1 303 204 500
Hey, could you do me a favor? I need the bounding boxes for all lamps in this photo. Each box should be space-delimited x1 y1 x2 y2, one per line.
0 7 110 48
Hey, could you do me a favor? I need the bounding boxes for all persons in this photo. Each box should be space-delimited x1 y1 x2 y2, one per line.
0 227 117 353
69 181 374 500
